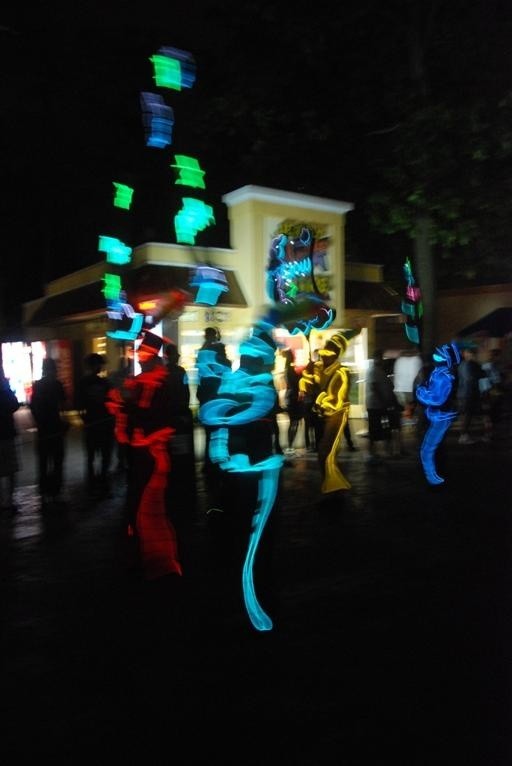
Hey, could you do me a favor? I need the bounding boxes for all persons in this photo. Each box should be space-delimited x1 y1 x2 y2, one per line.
1 373 22 514
31 358 71 507
